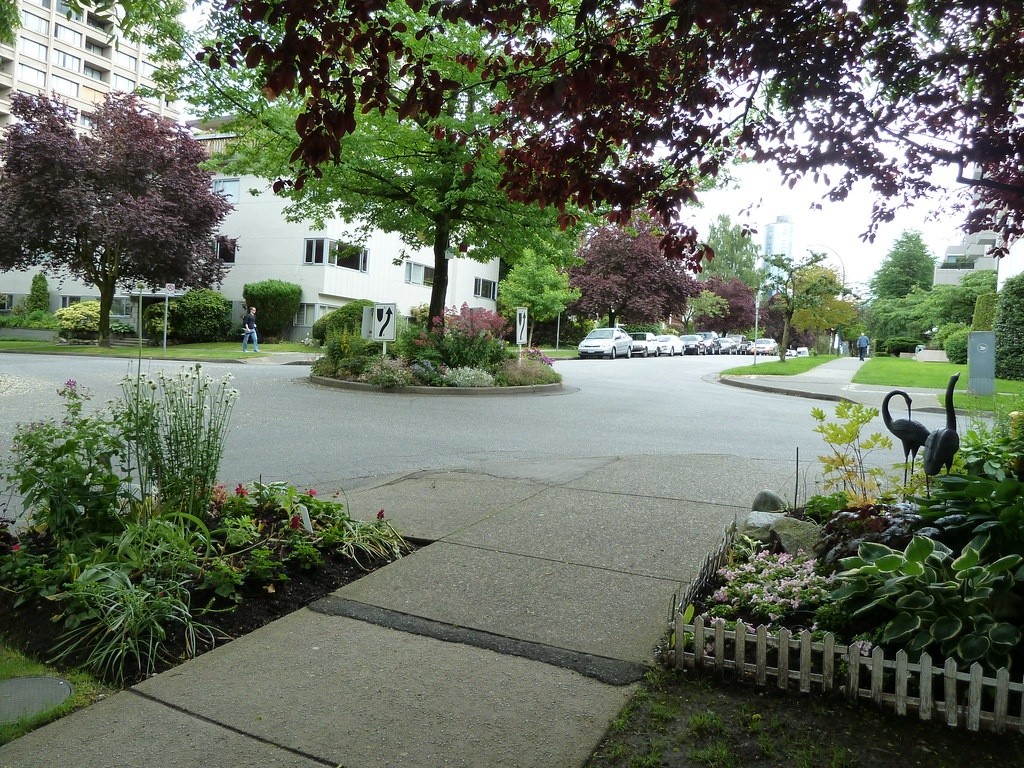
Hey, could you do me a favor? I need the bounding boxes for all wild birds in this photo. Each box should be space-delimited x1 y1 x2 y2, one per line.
881 372 961 494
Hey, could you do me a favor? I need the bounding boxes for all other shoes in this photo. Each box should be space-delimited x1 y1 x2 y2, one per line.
860 356 864 361
254 350 261 352
243 350 248 352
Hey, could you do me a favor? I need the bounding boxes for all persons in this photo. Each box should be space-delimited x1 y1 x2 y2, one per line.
857 333 869 361
243 307 261 352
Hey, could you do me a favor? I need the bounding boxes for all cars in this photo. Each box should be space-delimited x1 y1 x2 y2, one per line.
627 332 661 358
747 340 753 353
785 349 797 357
656 334 686 356
679 334 707 356
751 339 778 356
719 337 738 355
578 328 634 360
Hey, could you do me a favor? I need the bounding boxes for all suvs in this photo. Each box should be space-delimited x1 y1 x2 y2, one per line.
695 331 721 355
726 335 749 355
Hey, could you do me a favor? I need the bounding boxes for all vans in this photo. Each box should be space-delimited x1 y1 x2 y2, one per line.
797 347 809 358
